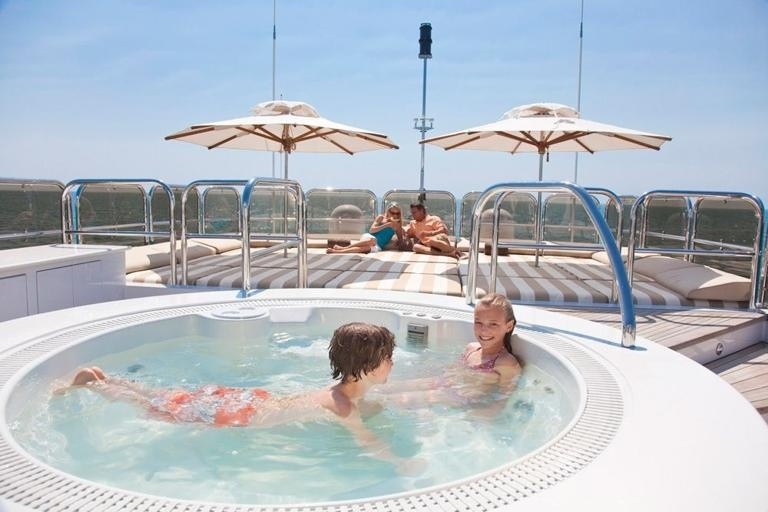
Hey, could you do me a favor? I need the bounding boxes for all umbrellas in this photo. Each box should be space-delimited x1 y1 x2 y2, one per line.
418 99 672 270
159 96 402 256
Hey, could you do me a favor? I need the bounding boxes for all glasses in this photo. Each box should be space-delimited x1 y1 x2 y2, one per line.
389 209 401 215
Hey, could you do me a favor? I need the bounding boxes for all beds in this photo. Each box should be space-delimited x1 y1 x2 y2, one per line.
125 239 748 308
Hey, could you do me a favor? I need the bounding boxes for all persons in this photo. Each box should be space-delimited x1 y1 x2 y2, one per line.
48 320 459 478
406 202 461 260
327 203 404 255
375 293 523 423
